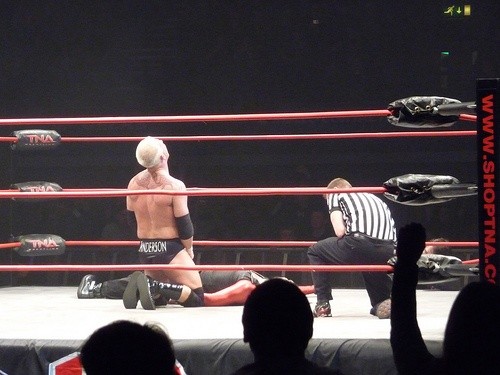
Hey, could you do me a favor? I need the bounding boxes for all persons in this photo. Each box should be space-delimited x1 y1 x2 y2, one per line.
99 200 452 283
390 222 500 375
228 278 337 375
306 178 398 319
77 270 317 307
79 320 176 375
123 136 204 310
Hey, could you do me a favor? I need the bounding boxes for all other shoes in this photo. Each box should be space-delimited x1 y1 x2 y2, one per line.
369 298 391 319
76 274 97 299
136 273 157 310
123 271 144 309
313 302 332 317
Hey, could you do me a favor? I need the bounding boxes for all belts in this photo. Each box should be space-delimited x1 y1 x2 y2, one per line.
352 232 395 245
